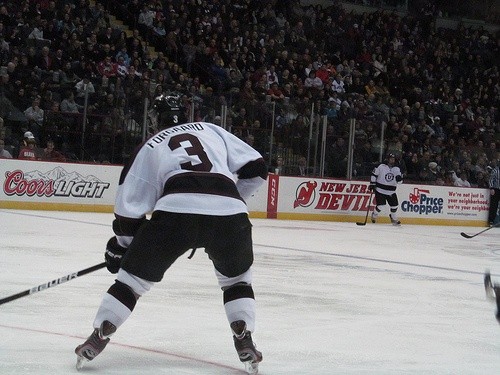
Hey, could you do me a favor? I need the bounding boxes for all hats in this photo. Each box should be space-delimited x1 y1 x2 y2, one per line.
428 162 438 174
24 131 34 139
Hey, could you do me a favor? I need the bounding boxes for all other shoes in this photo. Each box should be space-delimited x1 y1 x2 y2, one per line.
489 222 493 227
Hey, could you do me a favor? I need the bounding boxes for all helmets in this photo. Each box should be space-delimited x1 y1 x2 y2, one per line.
150 93 188 120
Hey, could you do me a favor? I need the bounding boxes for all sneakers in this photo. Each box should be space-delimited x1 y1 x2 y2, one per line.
371 212 376 223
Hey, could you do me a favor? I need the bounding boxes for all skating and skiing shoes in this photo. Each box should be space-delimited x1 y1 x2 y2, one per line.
233 331 264 374
391 220 400 225
75 329 111 370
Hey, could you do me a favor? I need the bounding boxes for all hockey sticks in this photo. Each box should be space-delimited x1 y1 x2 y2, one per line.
459 222 500 239
0 261 108 305
355 191 373 226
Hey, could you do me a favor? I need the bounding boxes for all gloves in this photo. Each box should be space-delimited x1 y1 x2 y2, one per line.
104 236 130 274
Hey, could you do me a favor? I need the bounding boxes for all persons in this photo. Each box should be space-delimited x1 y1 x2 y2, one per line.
76 103 268 364
488 159 500 228
368 153 403 223
0 0 500 189
